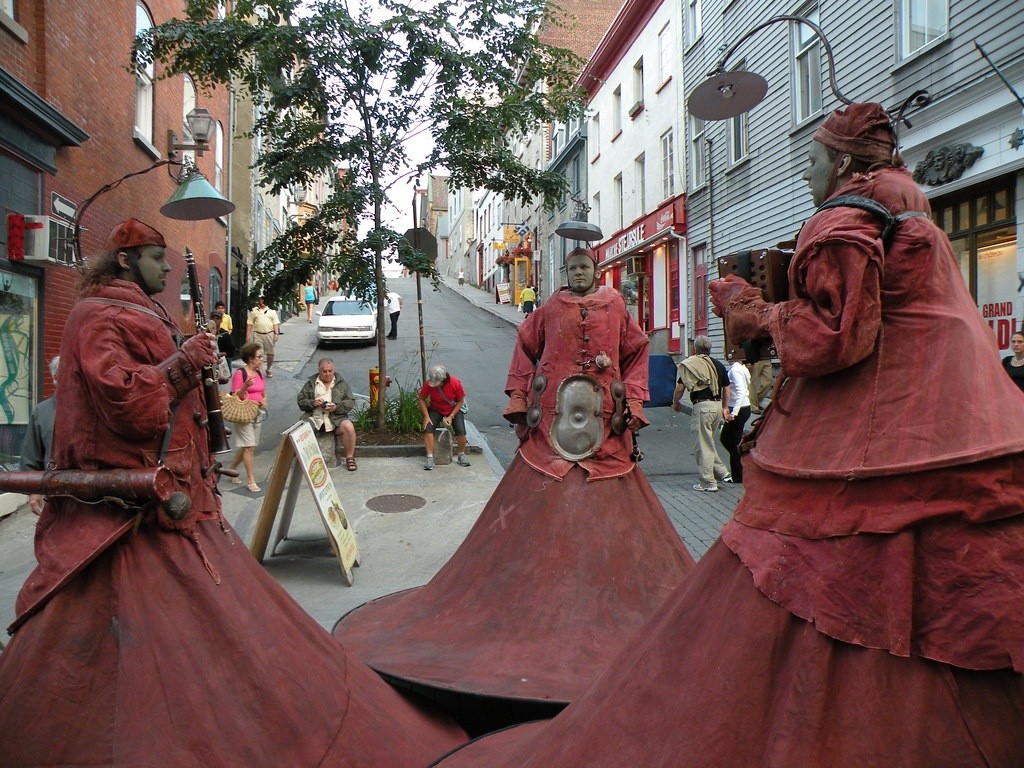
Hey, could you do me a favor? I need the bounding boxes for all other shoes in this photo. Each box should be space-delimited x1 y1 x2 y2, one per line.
759 405 765 410
266 369 272 377
386 335 397 340
524 313 529 318
752 409 762 415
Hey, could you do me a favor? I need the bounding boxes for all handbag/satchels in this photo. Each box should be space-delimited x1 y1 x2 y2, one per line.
533 303 536 311
518 301 522 312
217 355 231 381
221 388 259 422
449 399 468 414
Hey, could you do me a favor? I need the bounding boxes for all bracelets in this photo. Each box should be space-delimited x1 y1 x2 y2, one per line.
275 333 279 335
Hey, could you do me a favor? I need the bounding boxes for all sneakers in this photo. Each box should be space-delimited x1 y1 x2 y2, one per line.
457 453 471 466
424 456 435 469
723 474 733 483
693 483 718 492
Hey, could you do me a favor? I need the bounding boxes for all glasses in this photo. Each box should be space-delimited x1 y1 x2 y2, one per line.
254 354 264 359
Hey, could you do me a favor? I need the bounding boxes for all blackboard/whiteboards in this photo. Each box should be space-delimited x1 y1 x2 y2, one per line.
496 284 510 303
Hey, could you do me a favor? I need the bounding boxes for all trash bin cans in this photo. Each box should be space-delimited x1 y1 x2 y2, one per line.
330 280 337 290
328 285 333 290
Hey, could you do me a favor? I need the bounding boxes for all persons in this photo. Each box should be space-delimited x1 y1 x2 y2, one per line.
227 343 267 498
22 355 62 516
520 282 538 319
383 290 402 340
999 330 1024 394
331 246 699 704
305 281 320 323
418 365 474 470
206 301 234 378
0 215 472 768
432 103 1024 768
672 334 751 492
297 356 358 472
246 297 280 378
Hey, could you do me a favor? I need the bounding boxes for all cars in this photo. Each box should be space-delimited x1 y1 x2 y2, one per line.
314 295 379 346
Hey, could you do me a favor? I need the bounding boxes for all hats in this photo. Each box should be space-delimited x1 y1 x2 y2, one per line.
104 217 167 252
429 364 447 387
565 248 597 269
812 102 895 163
210 311 222 318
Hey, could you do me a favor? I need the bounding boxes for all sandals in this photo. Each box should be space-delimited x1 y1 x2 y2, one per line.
345 457 358 471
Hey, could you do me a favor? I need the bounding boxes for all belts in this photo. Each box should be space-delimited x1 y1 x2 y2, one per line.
253 330 275 335
693 398 721 404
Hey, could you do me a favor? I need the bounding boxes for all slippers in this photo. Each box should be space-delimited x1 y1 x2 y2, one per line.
230 476 242 484
247 483 261 492
